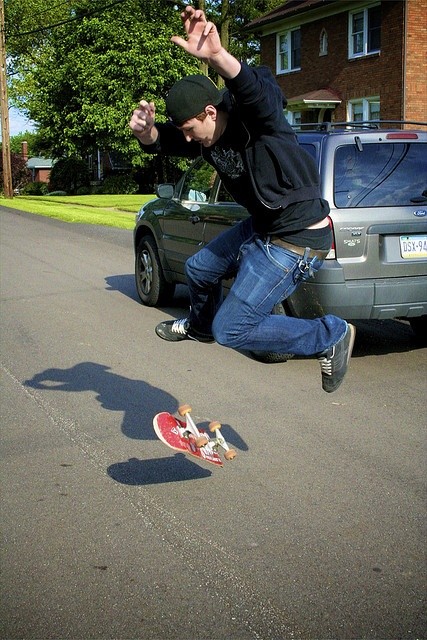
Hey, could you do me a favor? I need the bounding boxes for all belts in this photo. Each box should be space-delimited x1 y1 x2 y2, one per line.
269 237 330 260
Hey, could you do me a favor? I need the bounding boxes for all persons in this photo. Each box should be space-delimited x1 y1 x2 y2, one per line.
129 5 357 393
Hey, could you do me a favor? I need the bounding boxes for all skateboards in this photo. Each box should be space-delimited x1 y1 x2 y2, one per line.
152 405 236 467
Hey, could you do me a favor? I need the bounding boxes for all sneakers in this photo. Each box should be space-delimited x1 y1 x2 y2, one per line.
155 316 218 343
319 322 358 393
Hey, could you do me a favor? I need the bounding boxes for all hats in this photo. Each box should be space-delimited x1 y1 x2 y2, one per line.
168 74 228 129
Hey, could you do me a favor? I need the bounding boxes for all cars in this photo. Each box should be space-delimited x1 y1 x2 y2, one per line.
135 120 427 362
